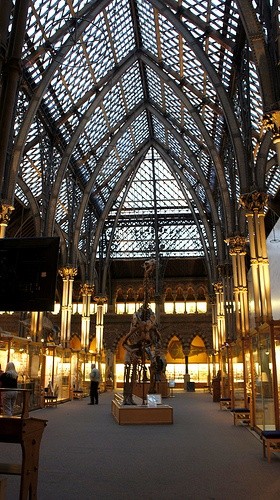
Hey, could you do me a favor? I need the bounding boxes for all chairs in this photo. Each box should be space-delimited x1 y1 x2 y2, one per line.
231 396 250 426
44 388 57 407
220 390 231 410
73 385 84 400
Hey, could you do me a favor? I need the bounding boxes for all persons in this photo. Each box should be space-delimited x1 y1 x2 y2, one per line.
148 351 164 382
0 363 5 409
88 363 101 405
0 362 18 416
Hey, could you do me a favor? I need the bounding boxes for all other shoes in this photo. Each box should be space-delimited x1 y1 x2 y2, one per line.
88 401 95 405
94 401 99 405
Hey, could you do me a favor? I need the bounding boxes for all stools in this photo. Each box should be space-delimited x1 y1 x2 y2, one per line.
262 430 280 464
